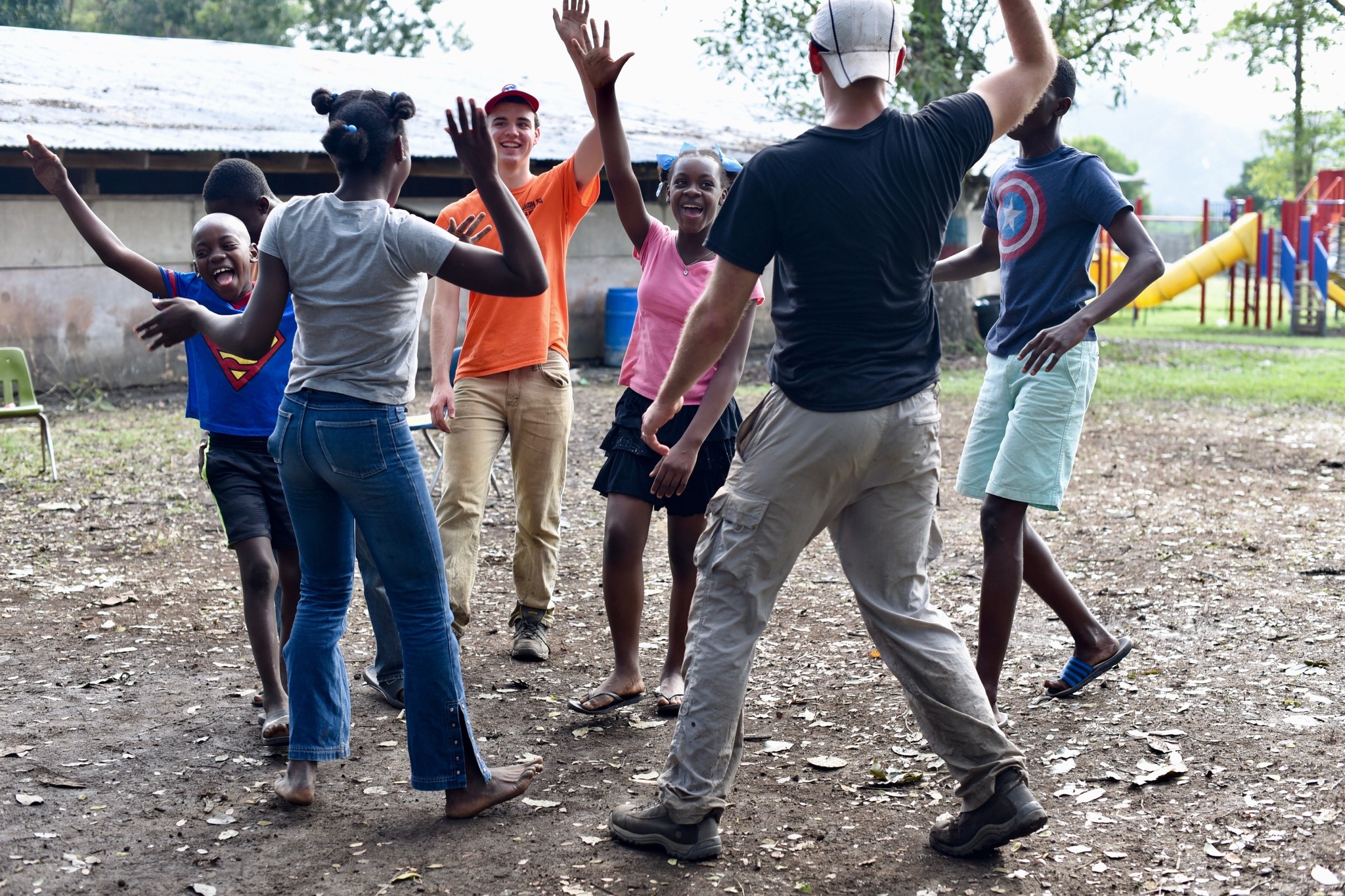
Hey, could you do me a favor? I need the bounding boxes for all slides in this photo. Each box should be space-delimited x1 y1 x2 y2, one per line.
1122 212 1258 308
1327 279 1345 308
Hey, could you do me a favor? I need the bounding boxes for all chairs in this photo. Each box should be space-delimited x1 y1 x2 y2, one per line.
0 347 58 483
405 347 500 495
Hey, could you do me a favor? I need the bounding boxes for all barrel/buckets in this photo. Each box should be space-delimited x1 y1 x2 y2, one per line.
604 287 639 367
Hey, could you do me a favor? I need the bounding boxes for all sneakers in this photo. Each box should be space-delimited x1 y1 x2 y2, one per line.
608 798 723 860
510 606 552 659
929 770 1048 857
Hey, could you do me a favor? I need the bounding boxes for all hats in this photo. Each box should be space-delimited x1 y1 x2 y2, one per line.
812 0 905 89
485 84 540 113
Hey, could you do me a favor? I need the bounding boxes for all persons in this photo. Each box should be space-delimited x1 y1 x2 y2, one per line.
136 85 548 818
570 18 765 722
428 1 604 656
606 0 1050 861
931 54 1165 726
200 159 404 710
22 133 298 749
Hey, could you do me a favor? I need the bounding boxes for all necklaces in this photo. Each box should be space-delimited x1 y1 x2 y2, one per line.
674 234 713 276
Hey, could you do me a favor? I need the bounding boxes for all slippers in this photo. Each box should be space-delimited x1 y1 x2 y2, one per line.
258 710 290 745
568 689 643 715
1045 637 1131 695
997 714 1008 727
362 665 404 711
655 692 684 714
252 689 263 705
